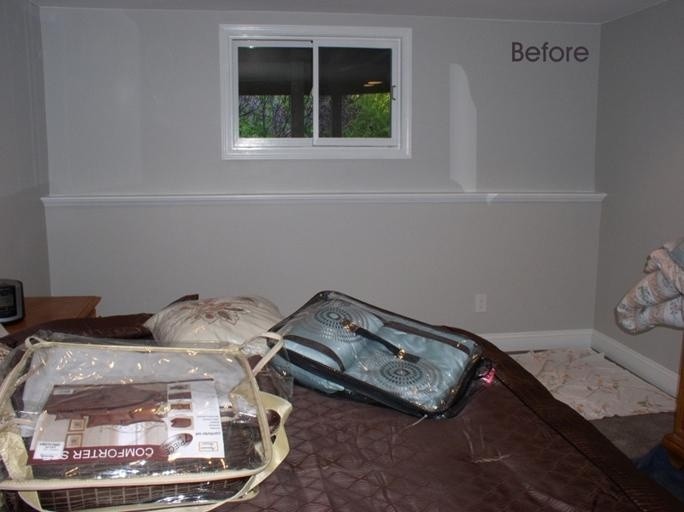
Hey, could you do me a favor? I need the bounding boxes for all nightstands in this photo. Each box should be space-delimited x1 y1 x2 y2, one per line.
1 296 101 334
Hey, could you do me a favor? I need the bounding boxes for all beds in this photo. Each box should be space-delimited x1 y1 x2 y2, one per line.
0 325 684 512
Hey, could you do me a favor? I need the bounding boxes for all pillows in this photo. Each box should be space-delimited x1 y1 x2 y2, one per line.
141 294 285 356
